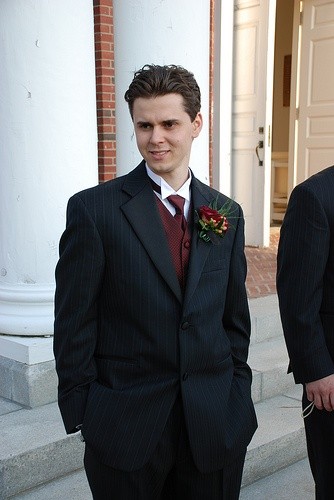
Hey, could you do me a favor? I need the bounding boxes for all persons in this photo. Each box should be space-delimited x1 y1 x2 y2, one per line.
276 164 334 500
53 64 258 500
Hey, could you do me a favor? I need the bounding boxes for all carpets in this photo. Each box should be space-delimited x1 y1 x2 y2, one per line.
242 229 282 300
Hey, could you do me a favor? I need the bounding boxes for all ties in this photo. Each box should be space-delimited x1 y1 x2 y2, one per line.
146 176 188 233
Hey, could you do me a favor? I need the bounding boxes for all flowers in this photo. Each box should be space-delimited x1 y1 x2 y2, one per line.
194 193 243 242
